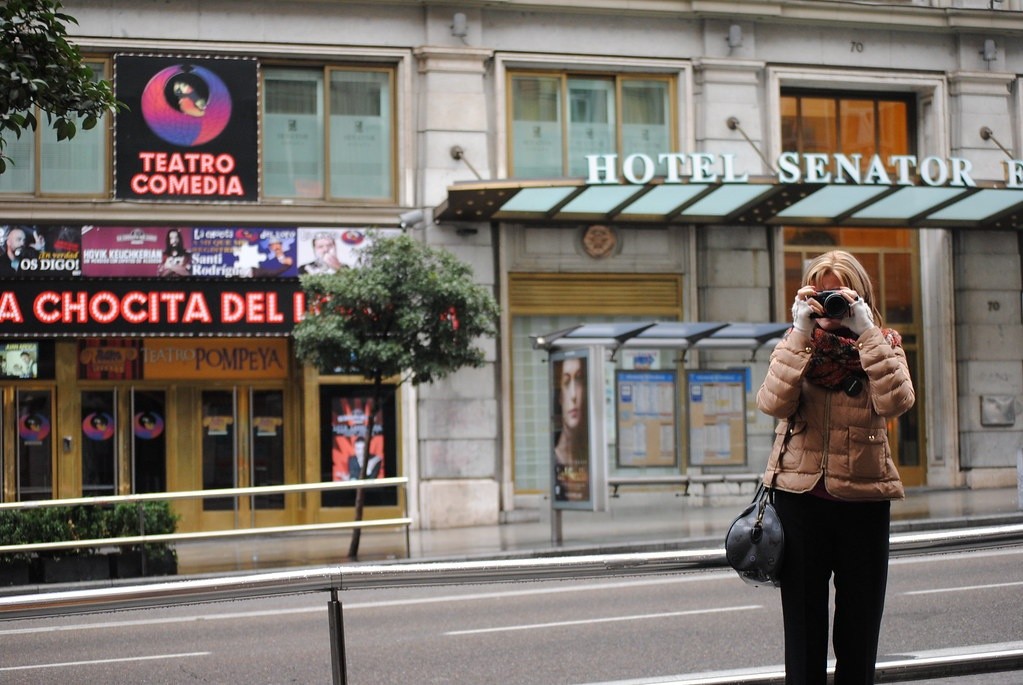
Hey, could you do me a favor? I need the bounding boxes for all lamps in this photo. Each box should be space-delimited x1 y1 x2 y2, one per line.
399 209 436 231
983 40 998 70
449 12 473 49
724 24 745 57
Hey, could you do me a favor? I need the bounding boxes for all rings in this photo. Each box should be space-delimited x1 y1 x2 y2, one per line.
854 296 859 301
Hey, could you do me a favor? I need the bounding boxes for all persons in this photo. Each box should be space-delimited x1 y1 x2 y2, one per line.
757 251 914 685
158 229 191 276
298 232 349 275
0 229 45 276
349 438 382 479
553 358 588 502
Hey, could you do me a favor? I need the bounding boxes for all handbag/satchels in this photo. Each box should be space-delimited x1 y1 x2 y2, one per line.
725 501 784 588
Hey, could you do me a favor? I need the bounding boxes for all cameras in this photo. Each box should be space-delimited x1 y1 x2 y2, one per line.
806 289 858 318
